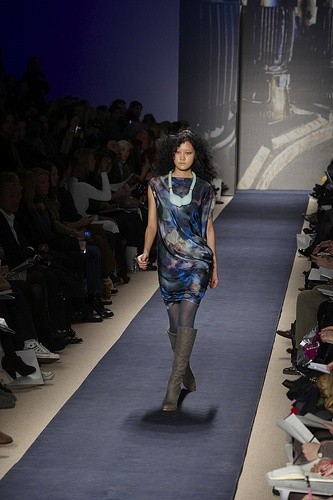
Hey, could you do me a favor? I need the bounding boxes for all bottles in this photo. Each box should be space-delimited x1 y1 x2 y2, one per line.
131 256 137 274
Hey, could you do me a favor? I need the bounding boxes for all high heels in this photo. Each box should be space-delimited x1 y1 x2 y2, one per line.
1 355 36 380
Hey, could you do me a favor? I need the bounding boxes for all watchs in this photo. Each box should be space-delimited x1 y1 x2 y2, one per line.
317 445 324 458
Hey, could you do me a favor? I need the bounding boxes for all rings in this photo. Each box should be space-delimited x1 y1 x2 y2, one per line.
321 333 324 338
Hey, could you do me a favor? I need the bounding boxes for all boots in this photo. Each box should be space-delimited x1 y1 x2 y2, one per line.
162 325 198 411
282 375 306 389
165 328 196 390
73 293 114 322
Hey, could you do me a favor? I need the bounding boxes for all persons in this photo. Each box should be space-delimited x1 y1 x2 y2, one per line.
0 98 229 445
276 158 333 500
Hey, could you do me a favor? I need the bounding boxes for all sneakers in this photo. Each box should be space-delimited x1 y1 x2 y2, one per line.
23 339 60 360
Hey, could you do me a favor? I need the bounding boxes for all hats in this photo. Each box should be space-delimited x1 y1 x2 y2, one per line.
95 148 117 167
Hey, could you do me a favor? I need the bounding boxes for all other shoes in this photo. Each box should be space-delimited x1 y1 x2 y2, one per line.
286 346 293 353
298 288 312 292
276 330 292 339
37 326 72 351
59 327 83 344
298 248 307 257
139 263 157 271
97 270 130 304
300 213 319 224
303 228 314 234
282 367 300 374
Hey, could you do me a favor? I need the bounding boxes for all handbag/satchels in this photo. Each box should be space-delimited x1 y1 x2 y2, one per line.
56 289 74 329
273 458 333 496
25 250 65 285
53 235 80 256
296 323 321 367
0 348 44 390
101 277 113 298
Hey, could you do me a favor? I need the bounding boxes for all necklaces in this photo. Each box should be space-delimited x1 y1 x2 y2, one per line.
168 169 196 206
